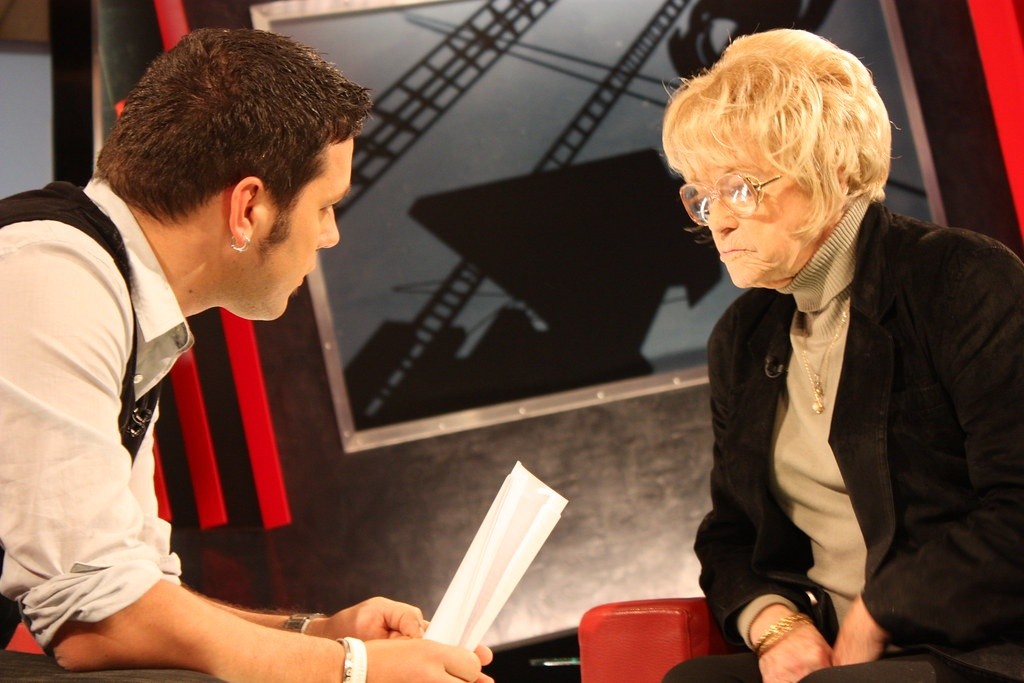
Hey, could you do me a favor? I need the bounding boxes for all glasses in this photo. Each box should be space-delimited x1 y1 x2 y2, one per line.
679 172 784 226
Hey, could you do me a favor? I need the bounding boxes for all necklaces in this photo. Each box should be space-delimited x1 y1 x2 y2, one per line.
802 307 849 413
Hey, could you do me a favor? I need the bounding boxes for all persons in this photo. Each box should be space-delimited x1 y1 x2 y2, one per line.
661 29 1024 683
1 27 494 683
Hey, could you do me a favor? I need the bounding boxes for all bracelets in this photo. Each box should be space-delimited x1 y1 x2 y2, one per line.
754 613 813 658
285 612 325 634
337 636 366 683
